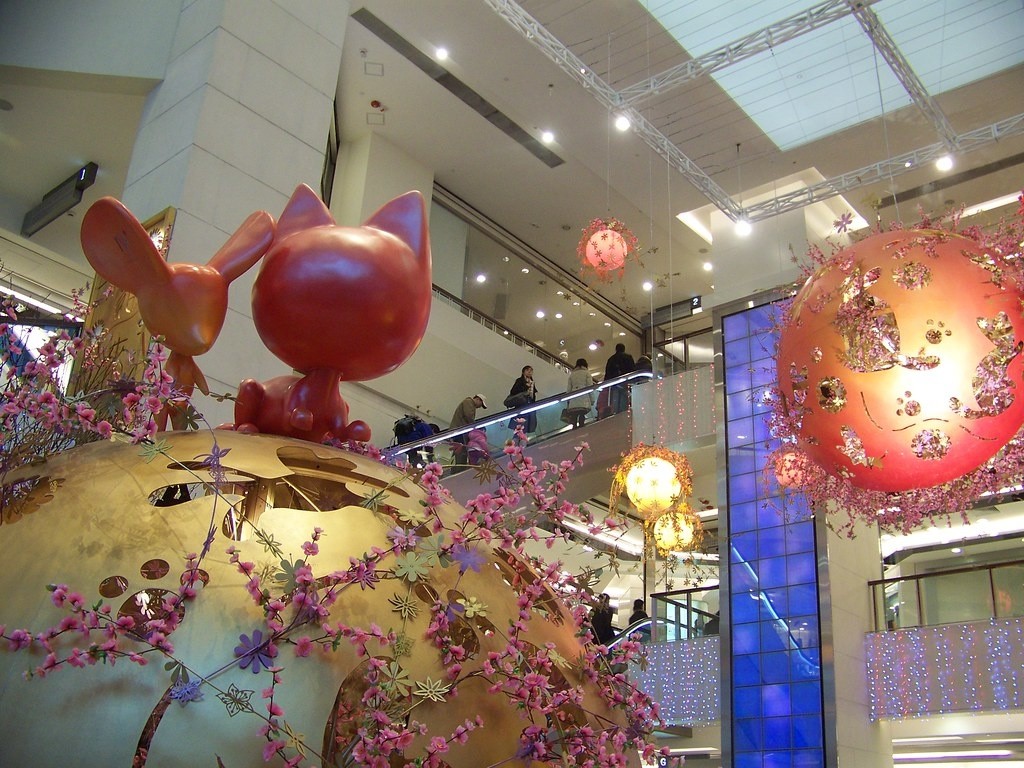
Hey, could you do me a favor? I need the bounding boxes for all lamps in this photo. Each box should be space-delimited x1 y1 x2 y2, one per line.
770 16 1024 504
620 120 705 559
587 28 632 274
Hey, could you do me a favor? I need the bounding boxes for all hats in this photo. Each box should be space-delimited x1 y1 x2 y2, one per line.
476 394 488 409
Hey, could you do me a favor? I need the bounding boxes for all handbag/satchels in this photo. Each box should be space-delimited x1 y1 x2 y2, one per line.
504 378 530 407
559 402 574 424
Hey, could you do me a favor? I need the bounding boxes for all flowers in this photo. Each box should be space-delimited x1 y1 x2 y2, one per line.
577 216 647 290
0 260 692 768
746 194 1024 544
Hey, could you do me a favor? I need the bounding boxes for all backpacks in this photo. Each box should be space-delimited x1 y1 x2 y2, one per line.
392 414 421 445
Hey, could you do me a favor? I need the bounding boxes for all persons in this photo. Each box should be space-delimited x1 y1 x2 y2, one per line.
450 394 490 474
508 366 538 446
566 359 595 430
396 423 440 468
629 599 651 642
695 611 720 637
604 343 652 414
584 593 615 646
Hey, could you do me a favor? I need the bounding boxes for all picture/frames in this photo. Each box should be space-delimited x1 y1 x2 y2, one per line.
64 205 177 444
